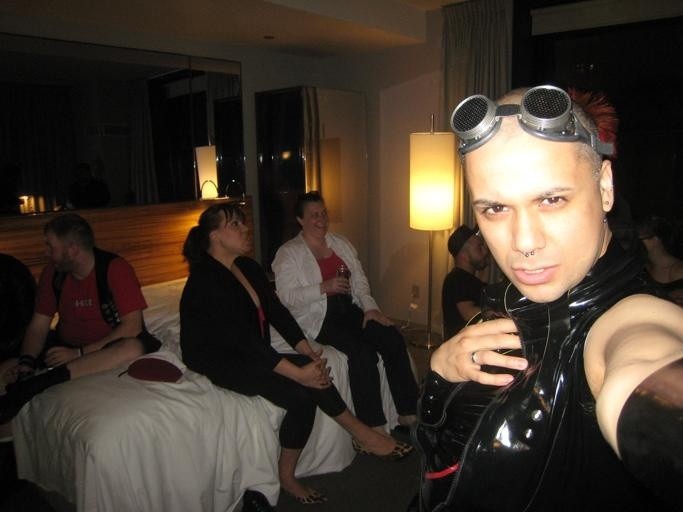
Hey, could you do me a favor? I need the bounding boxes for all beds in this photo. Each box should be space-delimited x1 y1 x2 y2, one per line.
13 302 349 512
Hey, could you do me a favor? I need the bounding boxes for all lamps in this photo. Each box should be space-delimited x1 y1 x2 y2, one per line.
191 133 219 199
409 113 459 351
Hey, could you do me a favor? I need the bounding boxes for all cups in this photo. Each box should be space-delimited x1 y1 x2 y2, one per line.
337 265 350 294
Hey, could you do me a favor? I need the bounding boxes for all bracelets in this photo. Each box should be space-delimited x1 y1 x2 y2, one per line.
18 354 38 368
77 347 82 357
80 347 85 357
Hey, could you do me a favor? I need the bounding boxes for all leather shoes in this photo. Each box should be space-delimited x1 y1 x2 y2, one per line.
351 436 412 459
280 483 329 505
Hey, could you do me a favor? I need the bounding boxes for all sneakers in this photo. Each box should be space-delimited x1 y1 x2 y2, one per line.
242 490 273 512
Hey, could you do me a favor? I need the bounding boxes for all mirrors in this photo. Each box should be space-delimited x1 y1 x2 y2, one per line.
0 32 246 216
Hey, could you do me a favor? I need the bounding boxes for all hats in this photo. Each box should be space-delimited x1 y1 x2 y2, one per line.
447 224 479 257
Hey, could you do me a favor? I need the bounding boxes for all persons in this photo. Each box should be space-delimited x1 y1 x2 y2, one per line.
176 200 410 504
410 83 682 512
271 189 426 446
0 252 41 362
1 210 160 427
633 209 683 305
438 224 502 342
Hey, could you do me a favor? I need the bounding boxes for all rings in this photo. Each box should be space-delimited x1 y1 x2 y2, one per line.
473 348 479 364
